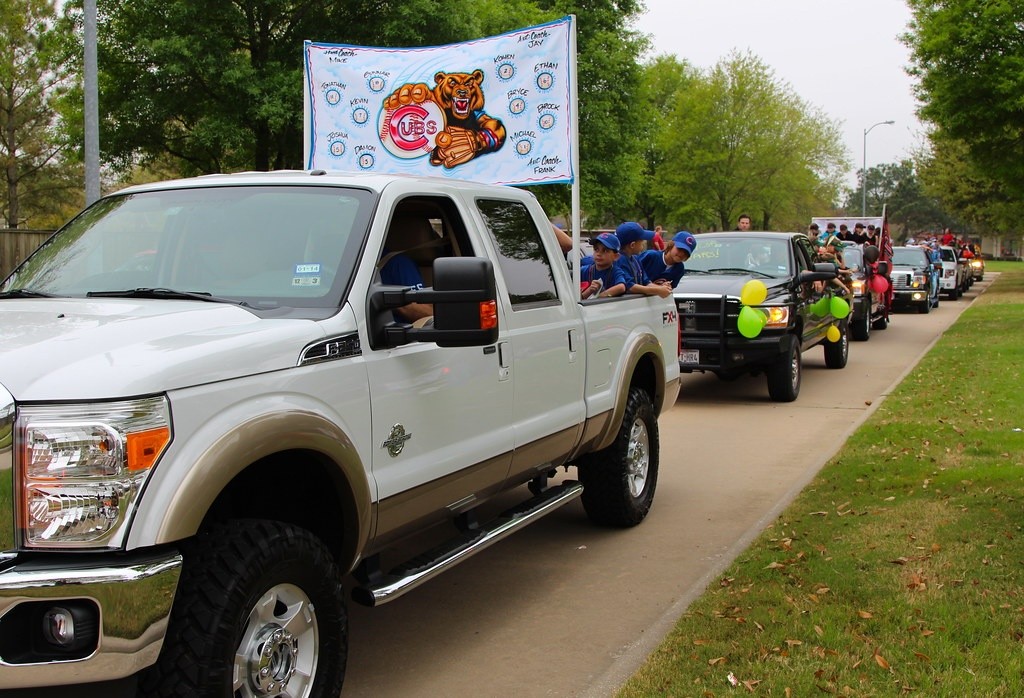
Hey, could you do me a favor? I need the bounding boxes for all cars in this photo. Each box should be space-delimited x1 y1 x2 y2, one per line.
567 234 594 269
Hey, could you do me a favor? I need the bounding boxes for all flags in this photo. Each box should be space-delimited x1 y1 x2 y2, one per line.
879 205 893 323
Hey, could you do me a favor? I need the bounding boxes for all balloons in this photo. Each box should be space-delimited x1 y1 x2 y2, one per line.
755 310 766 327
813 297 830 318
829 297 849 319
865 246 889 293
963 250 974 258
826 322 840 342
737 280 767 338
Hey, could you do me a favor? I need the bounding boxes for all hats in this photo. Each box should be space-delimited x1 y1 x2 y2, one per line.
930 237 937 241
906 238 915 244
588 233 621 252
918 241 928 244
840 225 847 231
809 223 819 230
868 224 875 230
827 223 836 228
671 231 697 255
615 221 656 242
856 223 866 228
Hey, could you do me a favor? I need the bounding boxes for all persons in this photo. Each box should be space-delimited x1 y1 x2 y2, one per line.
905 227 981 256
551 221 697 299
376 251 434 322
747 223 881 294
732 214 751 232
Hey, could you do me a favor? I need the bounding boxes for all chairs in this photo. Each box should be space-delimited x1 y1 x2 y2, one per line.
388 216 448 288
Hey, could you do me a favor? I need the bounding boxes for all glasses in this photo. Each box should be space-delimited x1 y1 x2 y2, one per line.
813 233 818 236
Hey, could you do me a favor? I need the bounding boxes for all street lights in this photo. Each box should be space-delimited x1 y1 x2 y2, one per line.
862 120 896 217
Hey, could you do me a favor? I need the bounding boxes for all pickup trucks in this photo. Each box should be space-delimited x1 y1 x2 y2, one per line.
836 238 890 340
888 244 943 314
0 168 682 697
671 231 854 404
936 250 984 301
